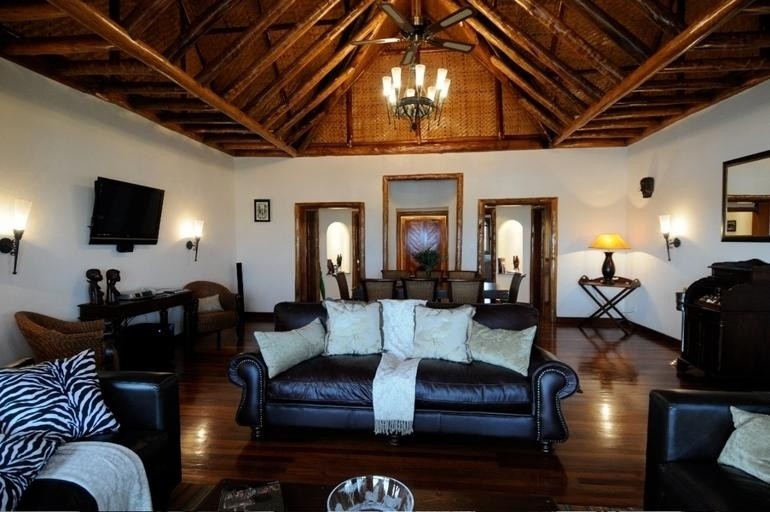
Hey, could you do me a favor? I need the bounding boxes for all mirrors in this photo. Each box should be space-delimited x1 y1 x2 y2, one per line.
382 173 462 278
720 149 769 242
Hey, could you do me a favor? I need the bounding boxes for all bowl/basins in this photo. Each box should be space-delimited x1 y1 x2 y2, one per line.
325 474 415 511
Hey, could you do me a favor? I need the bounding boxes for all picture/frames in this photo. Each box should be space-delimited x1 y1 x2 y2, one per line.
253 198 271 222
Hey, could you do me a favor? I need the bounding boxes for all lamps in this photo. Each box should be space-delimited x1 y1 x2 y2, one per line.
659 215 681 261
381 63 450 130
1 199 32 274
187 219 203 261
588 234 631 284
671 290 686 367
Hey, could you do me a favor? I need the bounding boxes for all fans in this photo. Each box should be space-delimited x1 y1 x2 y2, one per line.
351 1 475 69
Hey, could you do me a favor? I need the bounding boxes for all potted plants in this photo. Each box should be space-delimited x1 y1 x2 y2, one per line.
411 247 448 277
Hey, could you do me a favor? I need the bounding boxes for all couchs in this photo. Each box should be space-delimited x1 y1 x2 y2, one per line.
226 301 578 453
0 369 180 511
642 390 768 509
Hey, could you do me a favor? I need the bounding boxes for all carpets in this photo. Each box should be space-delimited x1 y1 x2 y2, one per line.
558 503 634 512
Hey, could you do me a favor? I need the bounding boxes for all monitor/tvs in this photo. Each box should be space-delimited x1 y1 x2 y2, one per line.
89 176 165 252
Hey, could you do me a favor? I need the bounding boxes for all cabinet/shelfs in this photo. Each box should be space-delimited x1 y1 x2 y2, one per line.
678 258 769 391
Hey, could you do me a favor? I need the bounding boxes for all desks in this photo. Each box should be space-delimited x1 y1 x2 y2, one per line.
577 275 640 336
76 292 198 354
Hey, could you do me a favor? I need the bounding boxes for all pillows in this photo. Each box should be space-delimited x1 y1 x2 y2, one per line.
469 323 537 377
323 299 385 354
0 348 120 442
252 317 329 379
414 305 475 365
380 299 426 355
197 293 222 314
0 430 58 511
716 404 770 484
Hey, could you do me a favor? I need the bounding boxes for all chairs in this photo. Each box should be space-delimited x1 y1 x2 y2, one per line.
15 312 109 368
331 268 526 303
184 280 237 347
